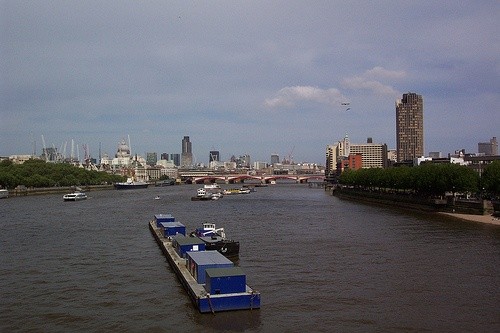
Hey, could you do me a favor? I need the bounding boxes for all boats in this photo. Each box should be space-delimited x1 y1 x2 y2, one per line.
113 176 149 189
189 219 240 259
191 183 257 201
63 192 88 201
